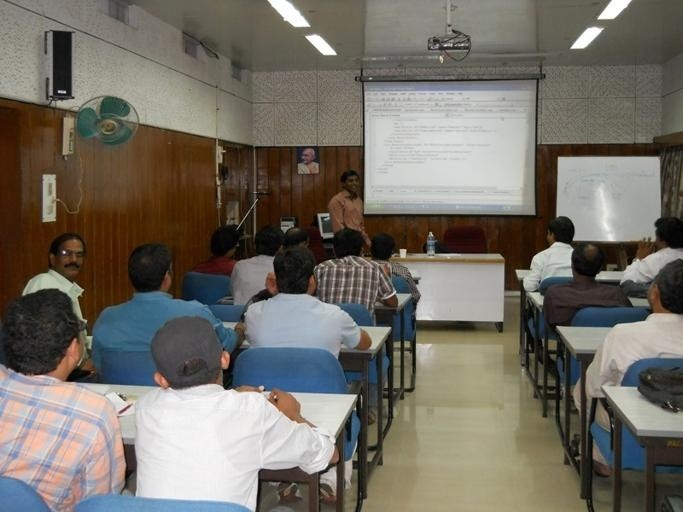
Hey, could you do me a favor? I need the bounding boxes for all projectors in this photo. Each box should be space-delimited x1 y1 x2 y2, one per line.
427 32 472 50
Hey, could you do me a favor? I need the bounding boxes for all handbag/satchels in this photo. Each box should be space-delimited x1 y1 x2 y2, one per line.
638 367 683 412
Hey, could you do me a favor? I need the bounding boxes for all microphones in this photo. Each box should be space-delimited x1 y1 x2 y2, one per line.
258 191 272 196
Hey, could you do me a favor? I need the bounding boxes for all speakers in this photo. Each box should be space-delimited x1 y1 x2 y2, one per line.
43 30 74 99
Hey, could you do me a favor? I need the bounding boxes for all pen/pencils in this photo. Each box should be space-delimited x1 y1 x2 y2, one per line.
118 403 133 414
274 395 279 401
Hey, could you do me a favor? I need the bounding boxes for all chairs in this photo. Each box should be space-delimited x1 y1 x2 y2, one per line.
0 272 412 512
555 308 647 385
590 359 683 472
528 275 573 339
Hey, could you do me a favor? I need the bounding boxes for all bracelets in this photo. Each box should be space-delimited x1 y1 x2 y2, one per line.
632 258 639 262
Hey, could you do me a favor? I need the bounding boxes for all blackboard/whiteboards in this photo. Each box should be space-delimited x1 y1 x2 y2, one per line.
554 155 663 245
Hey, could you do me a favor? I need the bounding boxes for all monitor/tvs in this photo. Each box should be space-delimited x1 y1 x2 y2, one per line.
317 213 342 239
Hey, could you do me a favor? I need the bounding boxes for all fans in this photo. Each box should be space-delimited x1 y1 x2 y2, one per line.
61 96 141 160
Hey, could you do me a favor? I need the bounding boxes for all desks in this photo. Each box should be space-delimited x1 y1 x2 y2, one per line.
601 385 682 512
514 269 623 367
556 326 615 499
392 253 505 332
527 292 650 418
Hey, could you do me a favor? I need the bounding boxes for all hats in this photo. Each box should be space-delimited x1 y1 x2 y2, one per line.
151 317 222 383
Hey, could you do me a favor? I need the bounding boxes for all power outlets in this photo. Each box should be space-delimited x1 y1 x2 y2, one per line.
42 174 57 223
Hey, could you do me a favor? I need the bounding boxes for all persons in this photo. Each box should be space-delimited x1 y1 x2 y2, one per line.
192 224 238 277
328 170 371 258
543 243 633 414
246 247 371 505
0 288 128 512
620 217 683 286
310 229 399 424
133 316 339 512
572 260 683 479
297 148 320 174
22 233 97 383
371 234 421 330
92 242 238 387
276 227 311 257
523 217 575 365
230 226 284 305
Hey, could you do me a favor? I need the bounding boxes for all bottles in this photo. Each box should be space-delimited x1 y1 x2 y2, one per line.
427 232 436 256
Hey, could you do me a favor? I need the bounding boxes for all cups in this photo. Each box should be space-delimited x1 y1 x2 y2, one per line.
399 249 407 258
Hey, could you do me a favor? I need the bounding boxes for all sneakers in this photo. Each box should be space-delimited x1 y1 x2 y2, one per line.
276 481 303 506
319 483 337 506
